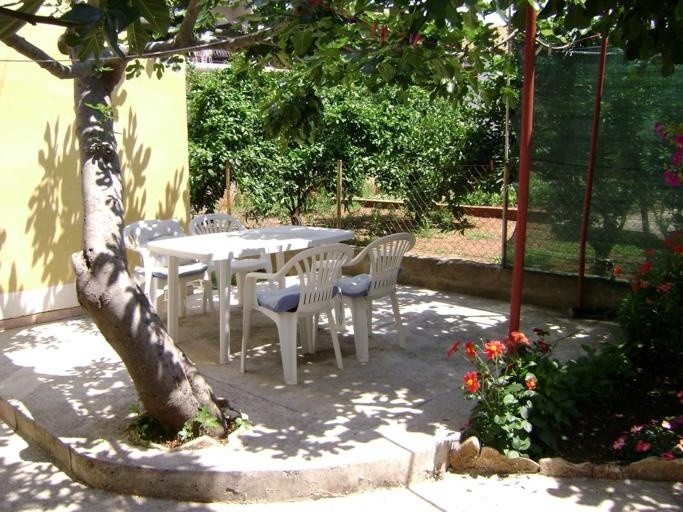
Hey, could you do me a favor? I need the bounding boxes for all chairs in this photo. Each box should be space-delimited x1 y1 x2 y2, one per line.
122 217 220 331
237 242 346 384
310 231 415 366
189 214 276 312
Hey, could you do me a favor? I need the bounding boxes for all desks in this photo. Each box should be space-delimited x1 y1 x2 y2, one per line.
146 224 355 364
